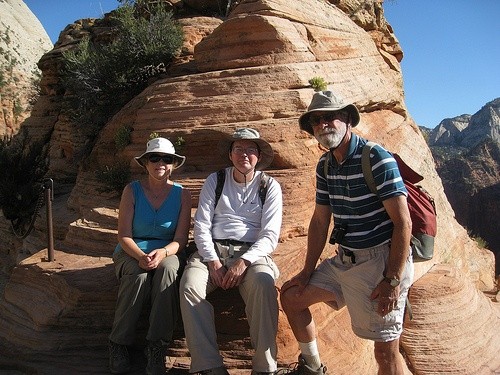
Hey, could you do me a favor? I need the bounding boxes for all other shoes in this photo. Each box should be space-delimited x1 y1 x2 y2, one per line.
274 353 327 375
191 367 230 375
251 370 274 375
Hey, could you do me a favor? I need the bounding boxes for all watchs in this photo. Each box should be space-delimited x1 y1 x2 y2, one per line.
381 270 401 287
163 246 170 257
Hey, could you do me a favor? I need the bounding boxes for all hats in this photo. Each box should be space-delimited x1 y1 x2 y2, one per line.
218 128 273 170
135 136 185 176
298 91 360 136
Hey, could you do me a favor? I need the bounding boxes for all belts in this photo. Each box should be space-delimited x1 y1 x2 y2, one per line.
212 239 255 248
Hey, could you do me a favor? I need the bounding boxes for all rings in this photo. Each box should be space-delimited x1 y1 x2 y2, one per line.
233 279 237 283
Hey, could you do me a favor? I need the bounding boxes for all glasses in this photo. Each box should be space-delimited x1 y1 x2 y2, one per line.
230 146 258 154
148 154 174 164
308 112 342 126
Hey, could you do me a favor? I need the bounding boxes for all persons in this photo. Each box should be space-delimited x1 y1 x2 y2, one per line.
179 126 285 375
107 137 190 375
280 90 415 375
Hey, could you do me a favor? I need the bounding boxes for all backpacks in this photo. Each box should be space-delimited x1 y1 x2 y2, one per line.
323 142 437 263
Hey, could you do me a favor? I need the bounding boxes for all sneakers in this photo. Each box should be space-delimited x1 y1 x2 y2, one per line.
141 340 167 375
108 340 131 374
